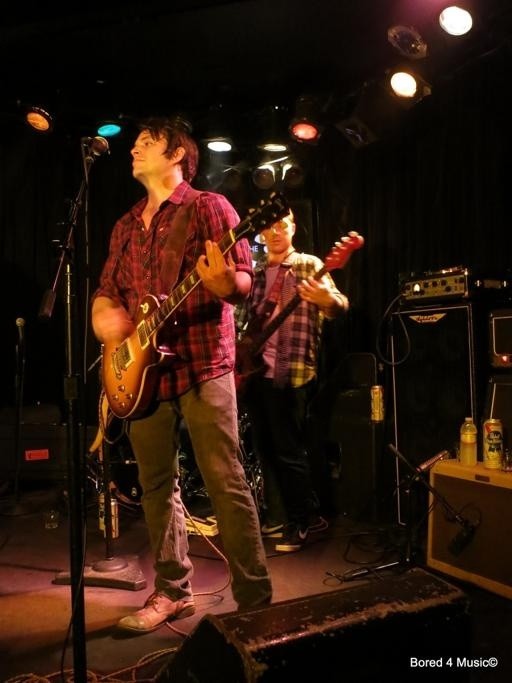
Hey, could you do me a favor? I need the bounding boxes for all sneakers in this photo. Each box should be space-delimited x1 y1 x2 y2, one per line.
261 515 328 552
116 590 196 633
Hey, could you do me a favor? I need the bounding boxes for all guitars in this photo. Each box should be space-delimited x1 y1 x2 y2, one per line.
236 231 365 397
100 188 289 419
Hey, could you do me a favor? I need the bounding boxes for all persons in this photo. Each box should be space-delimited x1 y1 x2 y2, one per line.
231 208 348 554
86 115 273 637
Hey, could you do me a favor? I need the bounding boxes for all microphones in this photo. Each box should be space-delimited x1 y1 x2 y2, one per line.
81 133 112 157
14 316 27 344
416 442 458 472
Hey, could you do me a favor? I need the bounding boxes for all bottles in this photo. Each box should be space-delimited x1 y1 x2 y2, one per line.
458 417 478 467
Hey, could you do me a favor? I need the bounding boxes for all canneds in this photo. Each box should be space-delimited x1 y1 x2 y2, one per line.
482 419 504 470
370 385 385 423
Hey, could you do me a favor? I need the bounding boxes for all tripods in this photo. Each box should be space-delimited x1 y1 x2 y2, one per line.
339 314 424 582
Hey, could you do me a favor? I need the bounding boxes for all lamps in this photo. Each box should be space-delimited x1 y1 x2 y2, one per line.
20 0 476 190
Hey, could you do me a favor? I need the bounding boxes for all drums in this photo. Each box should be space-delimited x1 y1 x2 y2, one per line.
102 425 185 504
